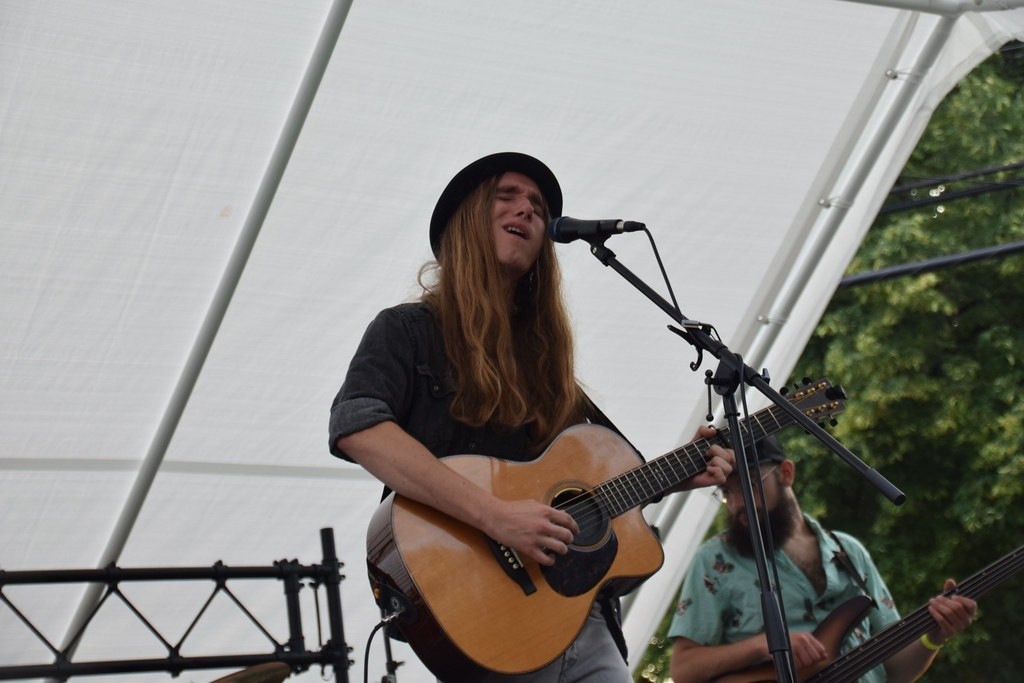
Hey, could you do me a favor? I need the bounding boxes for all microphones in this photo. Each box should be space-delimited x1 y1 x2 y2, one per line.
544 215 645 243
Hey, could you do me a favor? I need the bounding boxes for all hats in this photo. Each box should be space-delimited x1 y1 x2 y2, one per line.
429 152 562 259
728 434 784 477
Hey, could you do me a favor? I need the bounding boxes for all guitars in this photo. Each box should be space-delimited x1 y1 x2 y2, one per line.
363 373 849 682
712 542 1024 683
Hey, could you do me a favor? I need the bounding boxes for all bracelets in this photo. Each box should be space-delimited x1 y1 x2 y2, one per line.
921 634 944 651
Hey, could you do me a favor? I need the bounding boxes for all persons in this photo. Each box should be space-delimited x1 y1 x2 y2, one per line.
328 150 742 682
666 425 975 683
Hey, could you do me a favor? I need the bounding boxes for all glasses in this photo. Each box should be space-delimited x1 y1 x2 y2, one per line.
712 464 782 505
